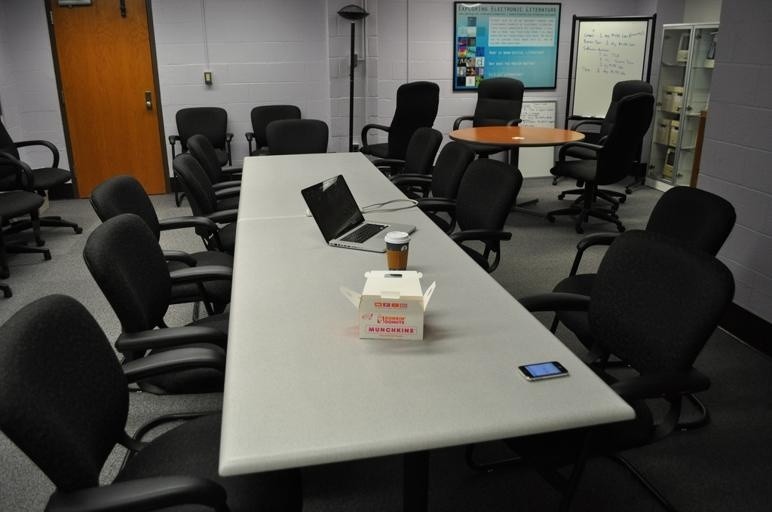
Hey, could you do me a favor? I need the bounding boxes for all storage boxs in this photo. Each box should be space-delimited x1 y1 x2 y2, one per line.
669 31 699 66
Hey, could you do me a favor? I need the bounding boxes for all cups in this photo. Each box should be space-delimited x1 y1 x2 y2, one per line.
383 230 411 276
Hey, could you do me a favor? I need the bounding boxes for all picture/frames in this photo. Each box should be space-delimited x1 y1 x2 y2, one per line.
448 1 563 93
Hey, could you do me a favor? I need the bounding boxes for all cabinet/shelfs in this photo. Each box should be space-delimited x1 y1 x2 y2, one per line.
639 19 721 193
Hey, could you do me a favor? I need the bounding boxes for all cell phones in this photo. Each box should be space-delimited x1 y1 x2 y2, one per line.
518 361 568 381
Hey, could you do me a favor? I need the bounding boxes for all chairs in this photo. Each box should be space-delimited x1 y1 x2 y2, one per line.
0 70 742 512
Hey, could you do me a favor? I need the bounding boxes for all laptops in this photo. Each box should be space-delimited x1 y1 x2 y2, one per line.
301 174 416 253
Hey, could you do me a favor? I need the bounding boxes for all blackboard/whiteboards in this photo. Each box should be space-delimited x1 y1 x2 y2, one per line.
571 19 649 120
509 100 557 179
